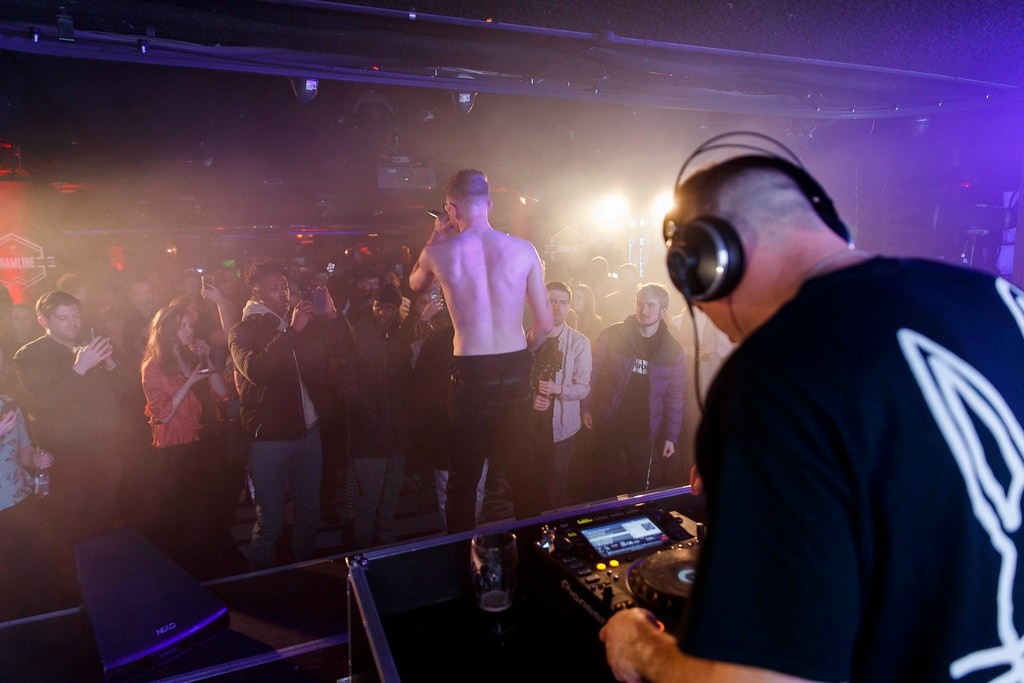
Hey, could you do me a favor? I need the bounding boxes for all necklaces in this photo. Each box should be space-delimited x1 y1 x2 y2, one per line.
800 250 870 282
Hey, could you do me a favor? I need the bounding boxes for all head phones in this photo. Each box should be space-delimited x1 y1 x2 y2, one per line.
664 130 855 304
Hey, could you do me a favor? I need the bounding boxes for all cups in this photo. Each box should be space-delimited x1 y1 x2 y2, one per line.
471 530 519 612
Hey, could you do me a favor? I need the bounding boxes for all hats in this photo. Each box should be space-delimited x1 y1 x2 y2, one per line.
373 283 402 306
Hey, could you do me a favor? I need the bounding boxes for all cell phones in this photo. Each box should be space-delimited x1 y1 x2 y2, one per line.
202 276 213 300
90 326 107 353
199 368 217 375
428 280 440 300
0 399 18 422
298 288 325 314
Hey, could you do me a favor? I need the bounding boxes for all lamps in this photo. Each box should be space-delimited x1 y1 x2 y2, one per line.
451 90 479 114
290 76 320 103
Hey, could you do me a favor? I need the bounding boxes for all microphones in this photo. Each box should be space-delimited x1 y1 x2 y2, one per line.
425 208 441 219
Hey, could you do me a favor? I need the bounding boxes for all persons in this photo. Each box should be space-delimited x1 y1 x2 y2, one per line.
0 242 686 618
600 155 1023 683
408 169 554 537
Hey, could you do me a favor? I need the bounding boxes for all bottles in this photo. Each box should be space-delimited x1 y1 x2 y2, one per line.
35 446 50 498
540 366 551 399
